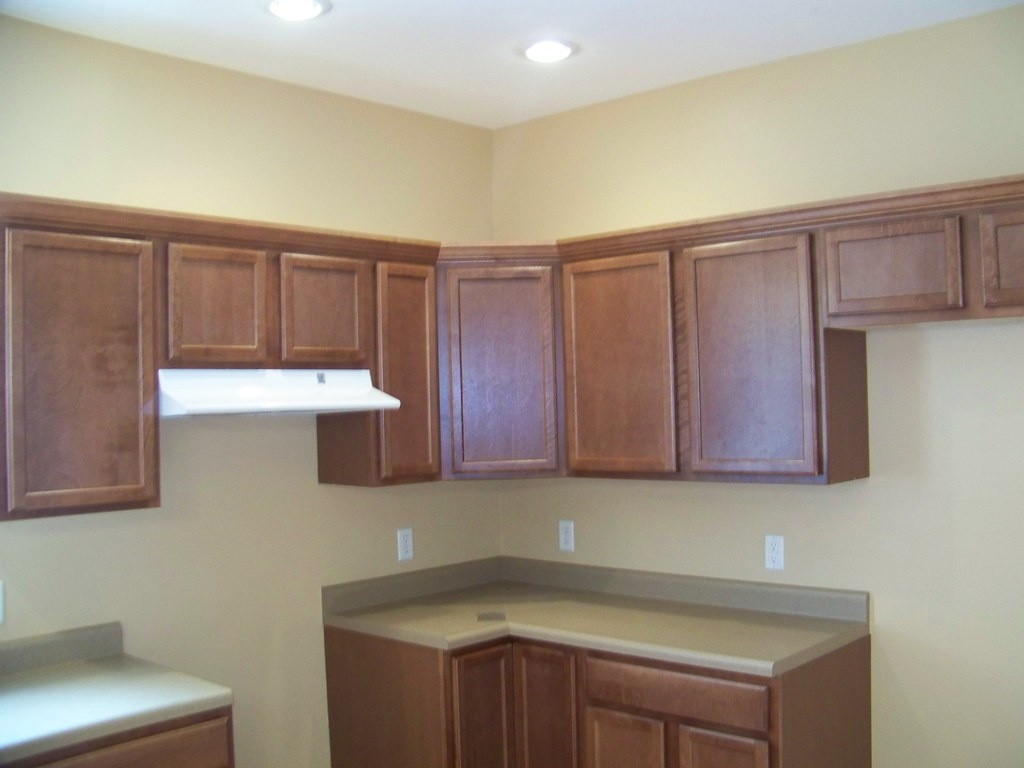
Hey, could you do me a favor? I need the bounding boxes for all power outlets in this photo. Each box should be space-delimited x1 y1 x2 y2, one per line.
397 527 413 560
558 520 574 551
766 535 784 570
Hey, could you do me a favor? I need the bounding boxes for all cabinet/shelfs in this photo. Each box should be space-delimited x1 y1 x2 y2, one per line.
0 705 236 768
325 631 871 768
0 174 1024 520
